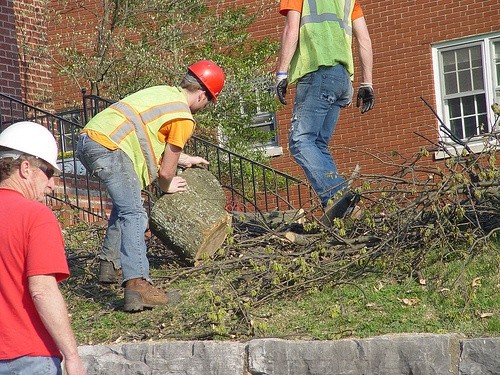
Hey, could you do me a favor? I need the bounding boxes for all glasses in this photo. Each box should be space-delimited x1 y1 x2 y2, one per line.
36 163 55 180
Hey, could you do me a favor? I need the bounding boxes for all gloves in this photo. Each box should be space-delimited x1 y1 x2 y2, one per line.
275 72 288 105
356 83 375 114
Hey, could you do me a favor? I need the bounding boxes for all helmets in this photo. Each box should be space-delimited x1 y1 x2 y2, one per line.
188 60 224 103
0 122 63 172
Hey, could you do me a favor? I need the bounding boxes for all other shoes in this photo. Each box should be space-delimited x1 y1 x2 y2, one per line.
318 190 360 228
99 259 120 283
124 278 183 311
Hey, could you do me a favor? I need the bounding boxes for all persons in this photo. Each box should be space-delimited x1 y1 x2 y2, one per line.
0 121 87 375
275 0 375 228
76 60 225 312
